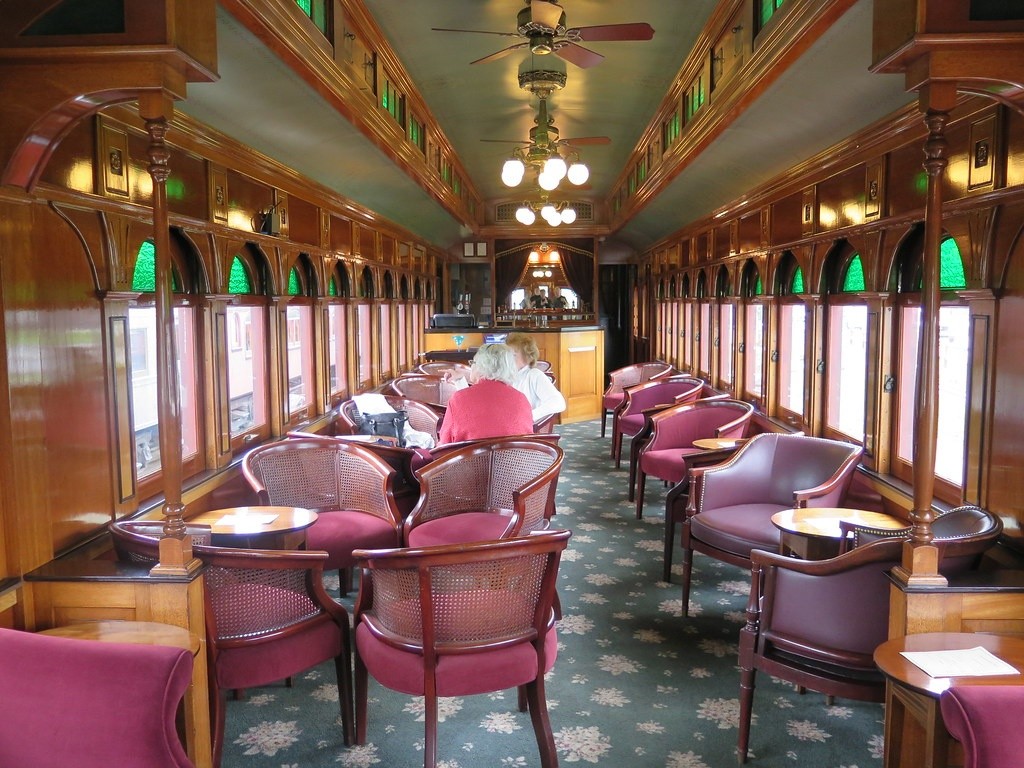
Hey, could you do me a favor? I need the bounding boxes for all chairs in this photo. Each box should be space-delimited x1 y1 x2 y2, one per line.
0 361 571 768
599 361 1024 768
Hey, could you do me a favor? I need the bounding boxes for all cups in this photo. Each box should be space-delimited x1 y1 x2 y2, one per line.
535 315 548 327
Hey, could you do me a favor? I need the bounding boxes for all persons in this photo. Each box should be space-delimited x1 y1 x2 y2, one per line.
551 286 569 310
531 288 552 307
438 331 567 422
436 343 533 448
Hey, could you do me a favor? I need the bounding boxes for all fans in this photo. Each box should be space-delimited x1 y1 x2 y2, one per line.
479 116 613 156
432 0 656 68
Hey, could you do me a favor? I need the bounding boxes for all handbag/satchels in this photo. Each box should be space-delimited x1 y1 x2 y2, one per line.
361 410 408 448
440 369 470 406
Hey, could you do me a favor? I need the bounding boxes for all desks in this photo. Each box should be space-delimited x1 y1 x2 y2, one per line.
874 633 1024 699
36 621 201 657
183 507 318 547
425 348 479 366
692 437 746 451
337 435 399 447
771 508 913 551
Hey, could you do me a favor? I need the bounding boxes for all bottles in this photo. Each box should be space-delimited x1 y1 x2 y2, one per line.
496 301 591 320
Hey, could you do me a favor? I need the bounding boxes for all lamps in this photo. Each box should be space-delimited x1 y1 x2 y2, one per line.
531 268 553 278
515 196 577 227
502 65 591 191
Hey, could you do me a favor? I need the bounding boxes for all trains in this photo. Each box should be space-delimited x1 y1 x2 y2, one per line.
127 305 370 469
